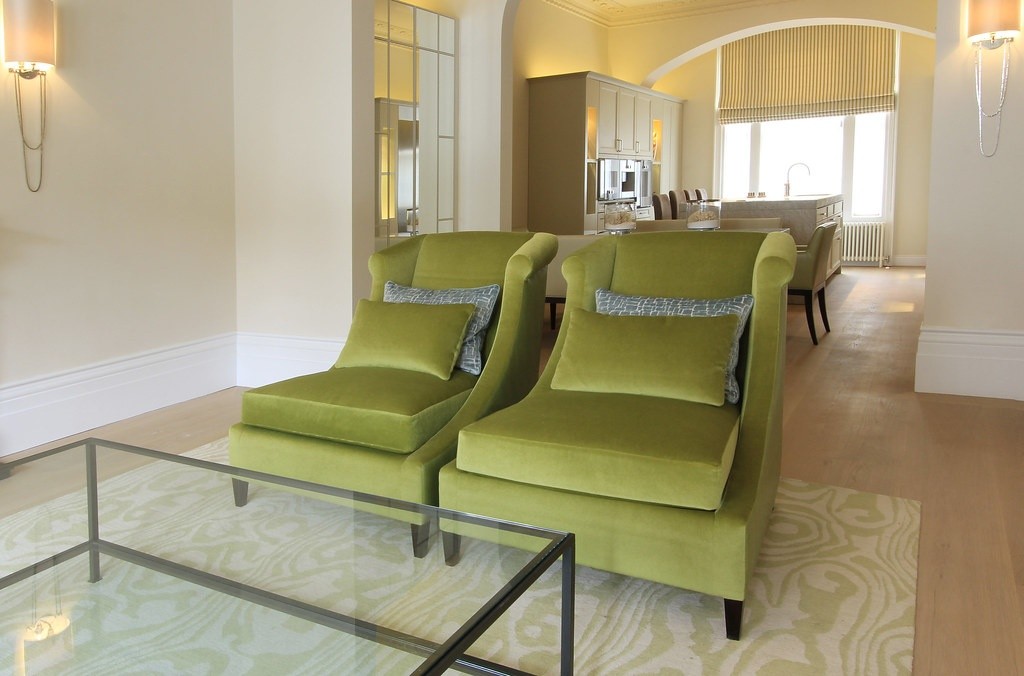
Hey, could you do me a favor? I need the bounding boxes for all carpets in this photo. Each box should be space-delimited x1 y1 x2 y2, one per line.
0 438 922 675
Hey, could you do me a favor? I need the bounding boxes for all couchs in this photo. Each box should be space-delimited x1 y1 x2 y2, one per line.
437 231 798 642
229 229 556 558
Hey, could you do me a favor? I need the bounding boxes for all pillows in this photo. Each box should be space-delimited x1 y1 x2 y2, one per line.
592 287 754 405
382 279 500 376
334 298 477 381
551 306 740 409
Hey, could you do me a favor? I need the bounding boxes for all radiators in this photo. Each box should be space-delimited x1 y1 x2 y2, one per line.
841 221 885 268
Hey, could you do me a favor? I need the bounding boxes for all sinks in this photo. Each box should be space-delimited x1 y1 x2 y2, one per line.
797 193 832 198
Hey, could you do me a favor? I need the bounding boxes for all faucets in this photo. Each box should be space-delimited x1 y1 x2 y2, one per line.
783 163 810 196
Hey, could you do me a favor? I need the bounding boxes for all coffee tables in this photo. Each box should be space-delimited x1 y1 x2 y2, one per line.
0 437 577 676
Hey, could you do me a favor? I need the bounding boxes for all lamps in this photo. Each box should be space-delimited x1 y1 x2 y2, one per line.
968 1 1021 158
2 0 57 192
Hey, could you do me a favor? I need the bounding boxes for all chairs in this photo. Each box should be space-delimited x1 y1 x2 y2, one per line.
652 194 673 220
695 188 707 199
787 221 840 345
683 189 697 202
635 219 689 232
719 217 782 230
669 190 687 219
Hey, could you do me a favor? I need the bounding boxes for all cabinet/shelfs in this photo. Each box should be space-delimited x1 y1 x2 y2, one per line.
526 71 688 235
720 194 844 305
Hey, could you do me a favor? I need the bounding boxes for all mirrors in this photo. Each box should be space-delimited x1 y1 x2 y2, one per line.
371 0 459 239
